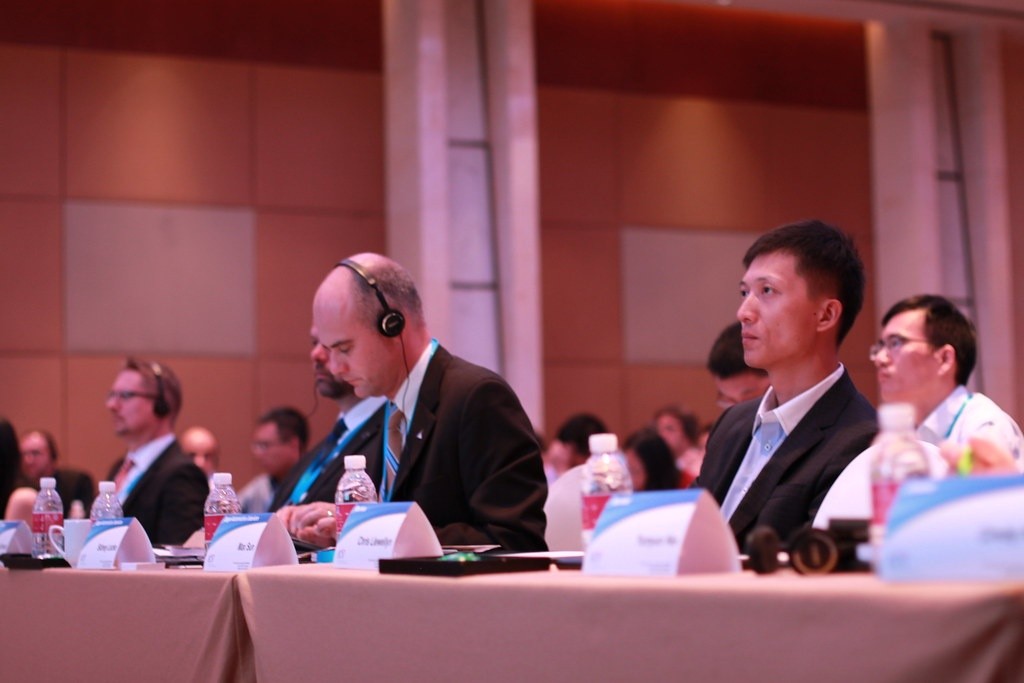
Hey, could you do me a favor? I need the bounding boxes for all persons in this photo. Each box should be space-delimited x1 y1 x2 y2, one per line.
0 414 42 534
178 424 217 480
267 254 550 574
539 403 715 564
235 406 309 512
89 357 209 547
689 219 879 570
711 323 771 412
871 293 1024 472
17 427 95 551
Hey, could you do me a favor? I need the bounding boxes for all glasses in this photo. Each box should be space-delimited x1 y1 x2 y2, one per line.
869 337 932 361
109 391 158 400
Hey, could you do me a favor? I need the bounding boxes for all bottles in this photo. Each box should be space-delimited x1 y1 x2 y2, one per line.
334 455 377 540
871 403 931 578
203 472 241 551
32 476 64 559
581 433 632 547
91 482 123 521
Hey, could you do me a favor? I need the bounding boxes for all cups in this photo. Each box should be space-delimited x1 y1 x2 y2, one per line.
48 519 90 568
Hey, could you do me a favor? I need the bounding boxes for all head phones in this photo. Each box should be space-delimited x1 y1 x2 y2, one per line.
336 258 405 336
744 519 876 575
129 354 171 417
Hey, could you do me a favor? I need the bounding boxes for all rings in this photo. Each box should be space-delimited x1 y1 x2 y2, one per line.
328 510 333 517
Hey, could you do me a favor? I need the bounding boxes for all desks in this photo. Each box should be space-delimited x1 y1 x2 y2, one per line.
235 562 1024 683
0 565 254 683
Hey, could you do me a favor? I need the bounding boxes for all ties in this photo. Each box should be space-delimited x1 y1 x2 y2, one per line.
114 458 134 496
386 409 405 494
